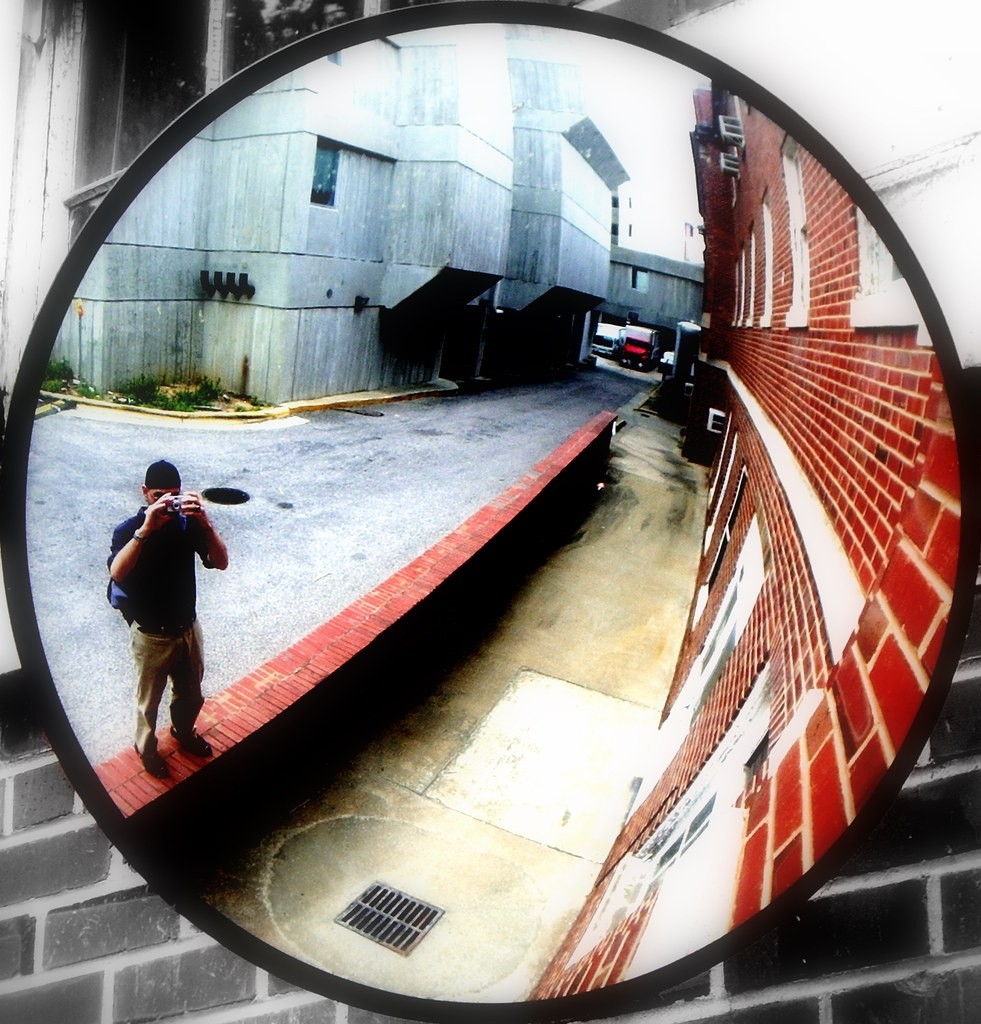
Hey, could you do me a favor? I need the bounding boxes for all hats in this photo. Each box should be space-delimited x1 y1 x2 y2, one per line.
144 460 181 489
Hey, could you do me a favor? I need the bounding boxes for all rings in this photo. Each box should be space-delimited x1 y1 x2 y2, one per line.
194 505 200 512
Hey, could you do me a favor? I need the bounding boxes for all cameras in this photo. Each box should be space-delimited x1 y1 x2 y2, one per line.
166 495 192 513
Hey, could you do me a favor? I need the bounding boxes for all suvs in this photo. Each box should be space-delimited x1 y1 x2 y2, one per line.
616 325 663 371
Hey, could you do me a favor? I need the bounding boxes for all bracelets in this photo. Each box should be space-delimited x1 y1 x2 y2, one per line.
133 529 148 544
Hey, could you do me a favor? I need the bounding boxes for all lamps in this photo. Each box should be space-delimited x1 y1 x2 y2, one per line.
355 295 370 313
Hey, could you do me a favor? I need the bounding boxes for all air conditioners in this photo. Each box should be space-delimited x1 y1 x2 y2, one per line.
719 152 739 176
707 408 727 434
720 116 743 145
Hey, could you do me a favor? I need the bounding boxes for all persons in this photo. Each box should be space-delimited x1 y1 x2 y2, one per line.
107 461 230 778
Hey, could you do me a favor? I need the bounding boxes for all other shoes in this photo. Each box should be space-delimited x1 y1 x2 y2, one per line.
134 739 170 777
170 726 212 757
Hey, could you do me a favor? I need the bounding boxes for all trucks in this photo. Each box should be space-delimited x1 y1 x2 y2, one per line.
590 323 623 360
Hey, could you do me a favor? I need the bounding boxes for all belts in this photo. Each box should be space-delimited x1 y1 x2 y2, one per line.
137 619 192 637
